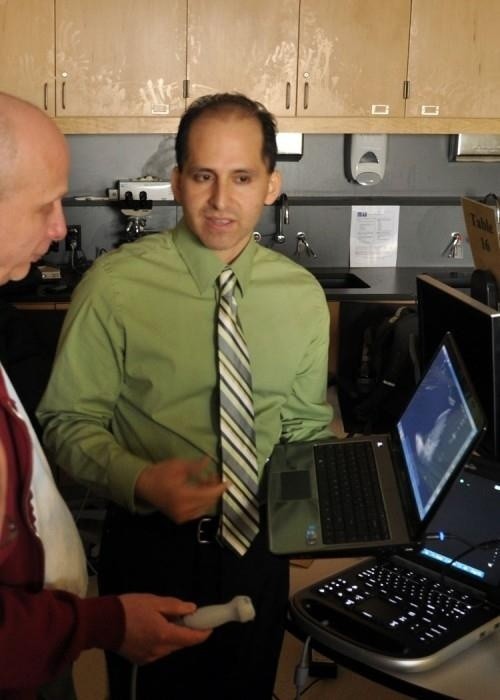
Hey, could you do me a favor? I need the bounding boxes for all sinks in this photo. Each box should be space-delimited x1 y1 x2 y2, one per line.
312 272 372 289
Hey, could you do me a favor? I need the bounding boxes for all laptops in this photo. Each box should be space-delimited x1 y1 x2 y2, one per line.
268 329 487 559
292 430 500 671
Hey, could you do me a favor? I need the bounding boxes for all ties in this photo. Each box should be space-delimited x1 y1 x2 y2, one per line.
215 271 262 558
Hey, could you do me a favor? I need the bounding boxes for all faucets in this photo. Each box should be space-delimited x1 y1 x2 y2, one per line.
277 194 290 236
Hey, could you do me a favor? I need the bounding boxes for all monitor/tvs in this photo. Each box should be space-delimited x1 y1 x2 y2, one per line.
415 274 500 463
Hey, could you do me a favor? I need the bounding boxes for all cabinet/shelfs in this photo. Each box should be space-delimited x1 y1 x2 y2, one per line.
404 2 500 123
0 1 188 120
188 2 410 119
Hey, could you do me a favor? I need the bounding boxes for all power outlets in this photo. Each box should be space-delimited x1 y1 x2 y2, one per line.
67 224 83 252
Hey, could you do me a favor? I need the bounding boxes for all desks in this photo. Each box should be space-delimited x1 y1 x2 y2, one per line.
282 431 500 700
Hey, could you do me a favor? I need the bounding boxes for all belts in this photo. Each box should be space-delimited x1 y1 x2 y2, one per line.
108 498 219 545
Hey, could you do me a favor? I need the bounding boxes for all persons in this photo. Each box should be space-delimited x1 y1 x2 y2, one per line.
0 90 219 699
36 89 342 699
